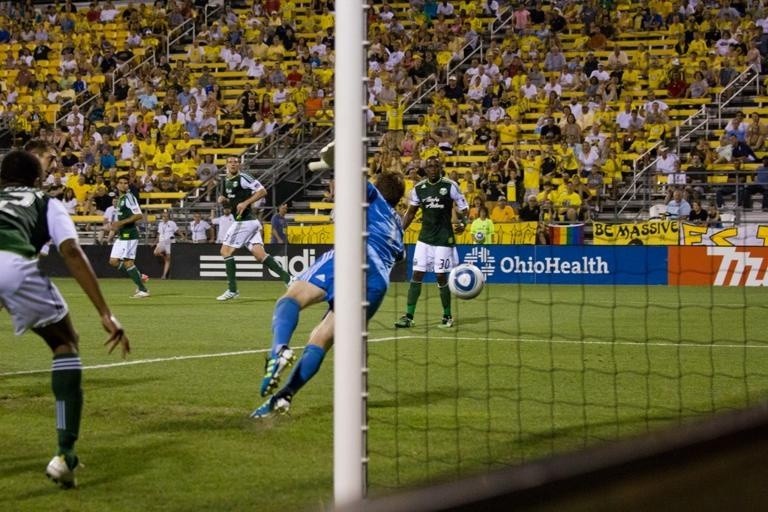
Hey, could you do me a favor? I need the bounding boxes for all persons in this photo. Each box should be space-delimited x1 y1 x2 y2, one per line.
153 208 185 281
0 151 130 488
396 156 470 328
1 2 767 244
217 157 294 303
250 171 407 421
109 176 152 300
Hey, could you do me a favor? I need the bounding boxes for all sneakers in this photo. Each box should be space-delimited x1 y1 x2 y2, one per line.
46 454 79 489
438 315 452 327
216 289 239 300
129 291 150 298
250 394 292 419
261 345 296 397
395 316 415 327
287 276 296 288
136 274 149 292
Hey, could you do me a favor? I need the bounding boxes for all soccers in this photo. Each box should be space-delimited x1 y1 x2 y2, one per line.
449 264 484 299
